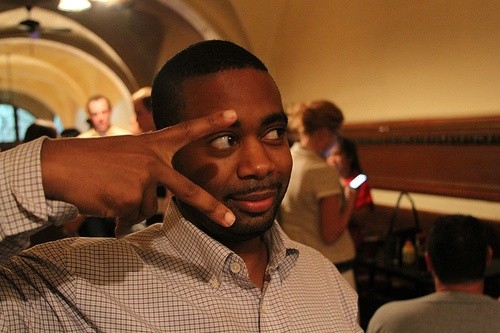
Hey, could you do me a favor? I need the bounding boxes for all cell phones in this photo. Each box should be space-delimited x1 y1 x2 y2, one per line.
345 173 368 192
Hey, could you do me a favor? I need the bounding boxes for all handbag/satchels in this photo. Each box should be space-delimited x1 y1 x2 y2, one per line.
381 191 428 265
